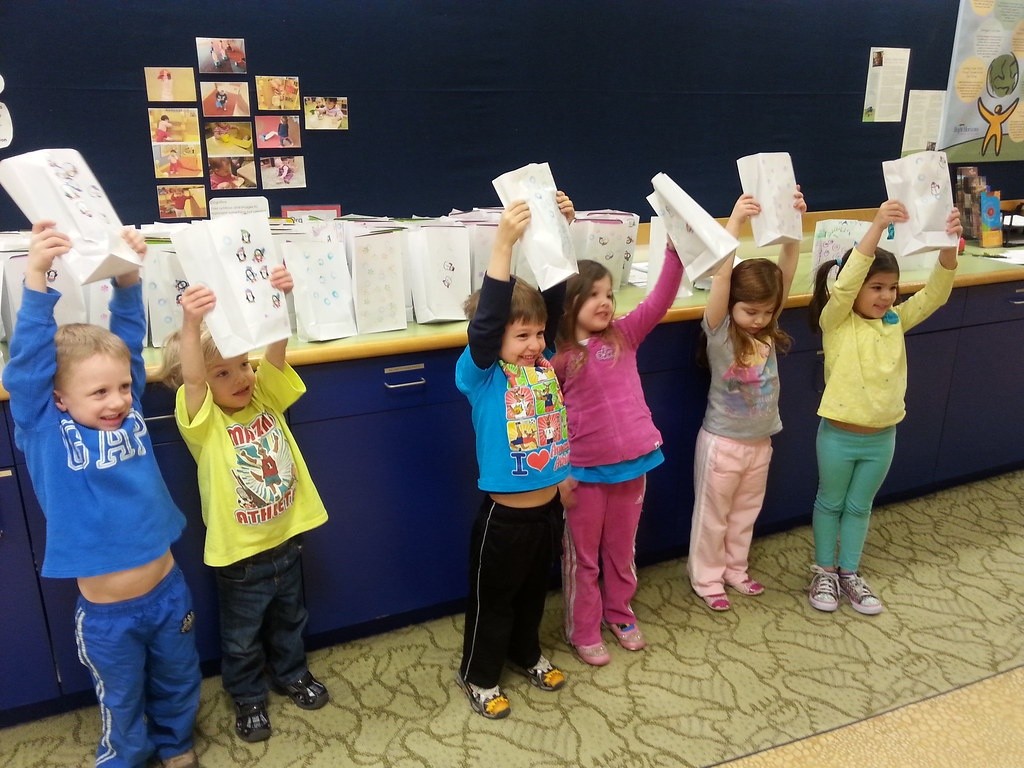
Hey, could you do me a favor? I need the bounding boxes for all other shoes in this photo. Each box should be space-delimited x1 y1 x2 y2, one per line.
611 619 647 651
158 747 197 768
575 642 610 666
704 594 730 613
726 577 765 597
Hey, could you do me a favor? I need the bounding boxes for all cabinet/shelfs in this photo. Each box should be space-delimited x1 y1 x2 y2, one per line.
0 276 1024 723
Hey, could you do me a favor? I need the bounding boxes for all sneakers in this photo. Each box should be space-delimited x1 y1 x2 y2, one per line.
506 654 563 689
803 566 841 613
269 670 329 710
459 670 510 720
837 566 882 616
236 697 272 742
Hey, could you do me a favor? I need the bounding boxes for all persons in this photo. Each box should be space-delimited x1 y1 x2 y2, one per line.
168 150 178 175
216 89 227 111
164 263 331 740
208 157 245 189
242 134 251 140
547 235 684 666
210 40 232 62
3 218 205 768
214 122 239 142
686 184 808 611
454 190 576 720
808 200 963 610
278 116 291 147
315 97 343 120
274 158 293 184
155 115 172 142
171 188 193 218
